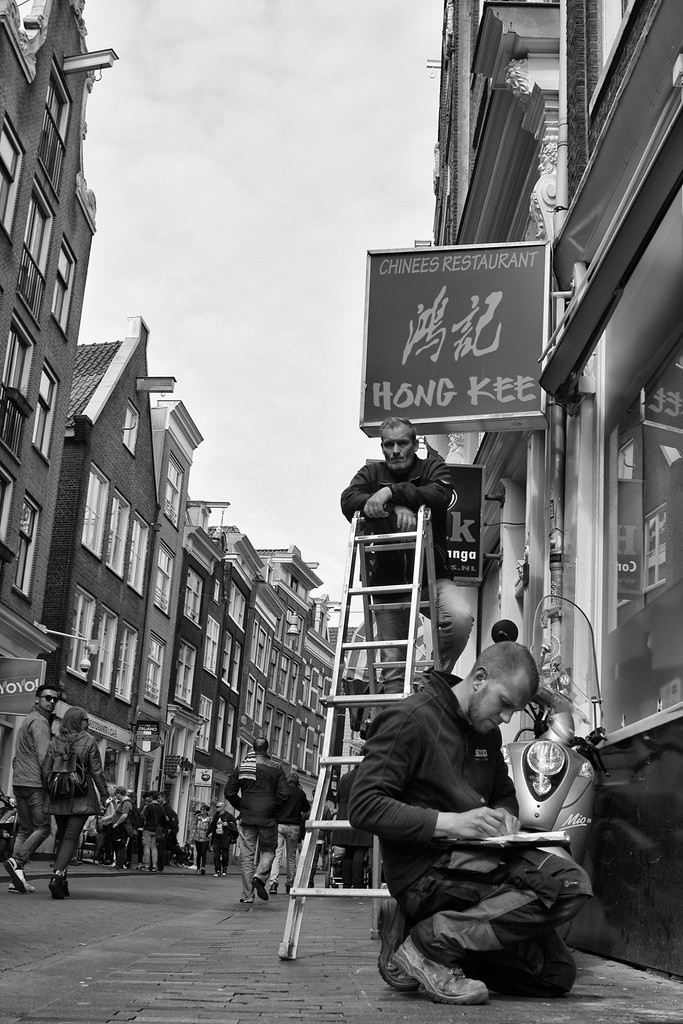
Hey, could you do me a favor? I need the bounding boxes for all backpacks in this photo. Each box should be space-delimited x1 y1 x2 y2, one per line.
120 799 145 830
46 744 88 806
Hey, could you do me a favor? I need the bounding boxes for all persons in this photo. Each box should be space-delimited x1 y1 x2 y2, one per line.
40 707 110 900
347 641 595 1006
341 416 473 691
224 737 292 904
268 760 373 894
3 683 58 892
69 787 239 877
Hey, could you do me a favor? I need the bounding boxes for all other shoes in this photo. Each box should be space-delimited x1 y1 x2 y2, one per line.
112 864 124 870
222 867 227 875
270 882 278 895
69 856 83 866
240 897 253 903
213 870 221 877
142 866 158 872
251 878 268 901
201 867 206 875
188 865 197 869
49 869 70 900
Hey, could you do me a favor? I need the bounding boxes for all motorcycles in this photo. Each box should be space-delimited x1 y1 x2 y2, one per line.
0 787 19 862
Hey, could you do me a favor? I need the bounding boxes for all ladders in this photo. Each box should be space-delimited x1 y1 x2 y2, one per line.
276 505 444 959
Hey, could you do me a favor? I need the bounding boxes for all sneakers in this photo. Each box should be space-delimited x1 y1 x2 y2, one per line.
8 883 36 893
377 899 421 991
392 934 489 1005
4 857 30 894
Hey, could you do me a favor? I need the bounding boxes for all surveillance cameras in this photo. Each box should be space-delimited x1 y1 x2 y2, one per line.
80 660 91 672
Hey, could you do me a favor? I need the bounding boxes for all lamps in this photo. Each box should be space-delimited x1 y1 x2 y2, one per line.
286 612 301 641
32 620 91 673
127 749 140 770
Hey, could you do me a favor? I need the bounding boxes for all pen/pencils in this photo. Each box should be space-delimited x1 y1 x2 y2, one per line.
479 796 494 809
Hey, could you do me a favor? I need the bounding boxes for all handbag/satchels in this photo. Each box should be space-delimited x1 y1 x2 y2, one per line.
156 826 168 842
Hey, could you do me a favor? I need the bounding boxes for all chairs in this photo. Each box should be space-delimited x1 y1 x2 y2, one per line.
78 830 98 863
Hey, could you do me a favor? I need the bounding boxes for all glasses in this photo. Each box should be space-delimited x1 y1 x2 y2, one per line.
40 695 59 703
